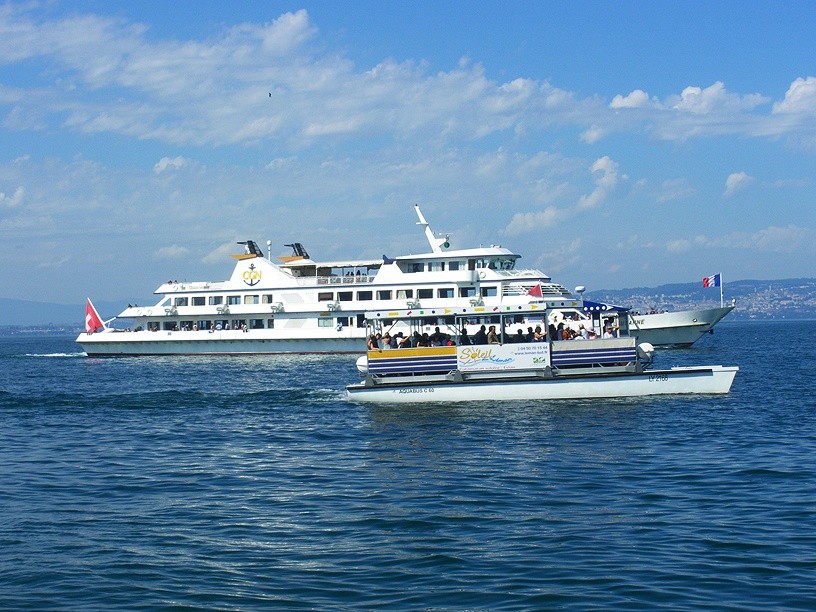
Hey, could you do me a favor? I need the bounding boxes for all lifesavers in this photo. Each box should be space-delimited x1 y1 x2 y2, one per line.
147 309 153 316
173 285 179 291
479 271 487 278
136 309 143 316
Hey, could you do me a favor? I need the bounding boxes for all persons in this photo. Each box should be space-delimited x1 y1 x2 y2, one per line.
91 269 670 355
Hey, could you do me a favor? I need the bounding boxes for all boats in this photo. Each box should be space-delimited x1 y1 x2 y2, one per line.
76 204 733 358
343 303 737 396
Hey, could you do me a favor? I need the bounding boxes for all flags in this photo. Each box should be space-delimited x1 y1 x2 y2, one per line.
85 299 102 334
702 272 721 287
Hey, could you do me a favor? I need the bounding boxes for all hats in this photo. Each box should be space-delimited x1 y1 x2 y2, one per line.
607 326 613 328
579 323 584 327
559 322 565 325
586 313 590 316
586 327 596 333
612 324 617 328
